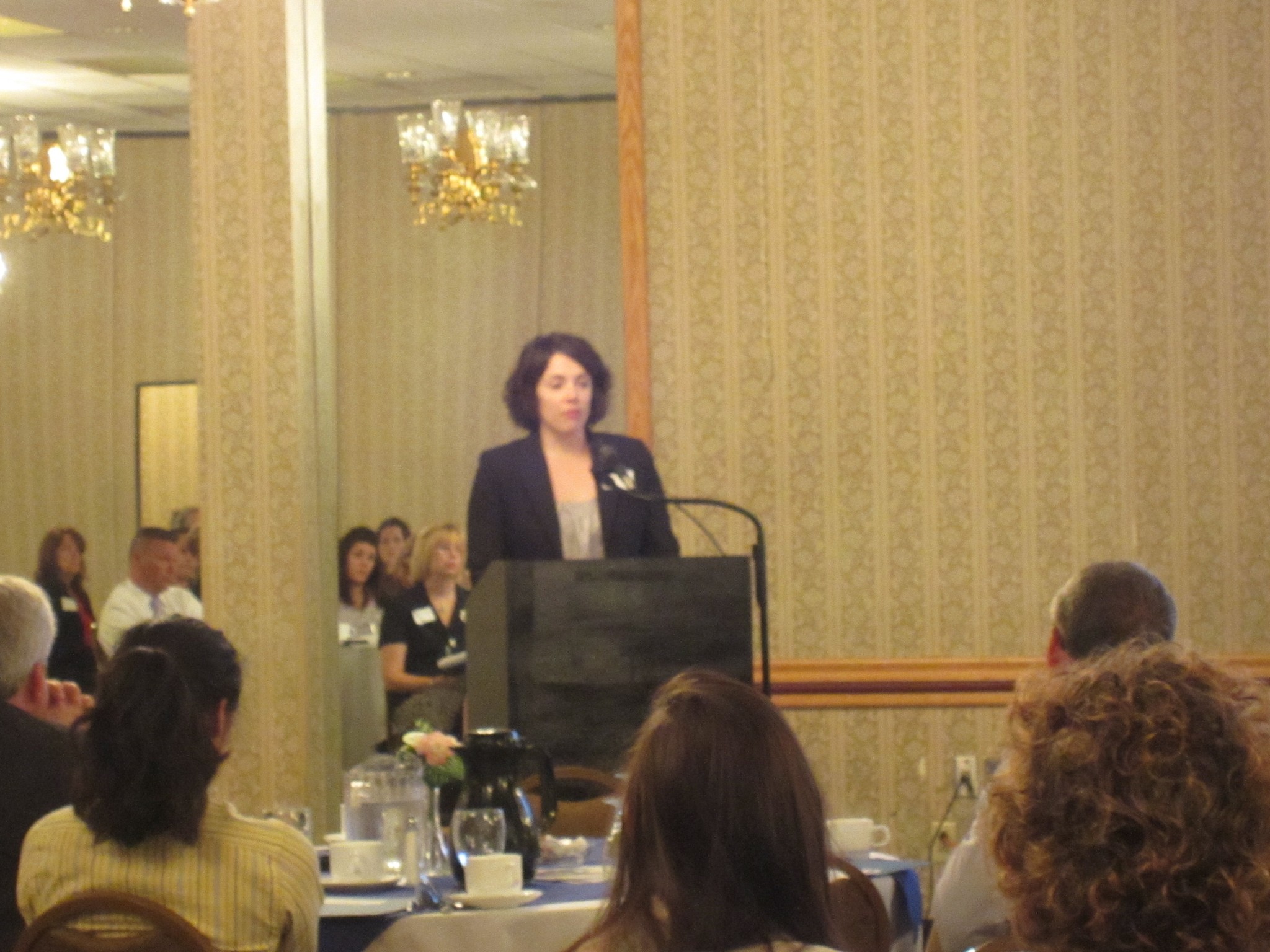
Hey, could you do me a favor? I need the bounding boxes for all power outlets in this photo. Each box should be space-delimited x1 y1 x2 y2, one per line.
956 752 981 803
928 821 956 866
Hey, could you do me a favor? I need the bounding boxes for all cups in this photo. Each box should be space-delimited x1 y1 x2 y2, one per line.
451 808 505 865
825 817 892 862
448 890 544 909
461 853 523 896
326 841 385 884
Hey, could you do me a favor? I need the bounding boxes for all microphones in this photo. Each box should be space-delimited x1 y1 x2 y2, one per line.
593 454 615 481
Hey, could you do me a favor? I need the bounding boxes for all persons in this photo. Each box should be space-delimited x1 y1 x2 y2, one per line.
987 644 1270 952
100 526 201 657
336 524 388 645
12 609 320 952
372 516 413 604
923 558 1190 949
376 522 471 693
29 528 108 700
0 574 93 938
465 334 682 585
168 508 196 529
173 534 199 591
565 667 867 952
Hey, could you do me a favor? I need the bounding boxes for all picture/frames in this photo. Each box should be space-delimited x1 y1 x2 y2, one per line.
134 377 199 539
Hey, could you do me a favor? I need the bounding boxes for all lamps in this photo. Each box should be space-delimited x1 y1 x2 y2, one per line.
389 93 546 229
1 108 122 246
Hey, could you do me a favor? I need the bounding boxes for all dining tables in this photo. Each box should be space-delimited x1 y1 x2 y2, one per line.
298 834 928 951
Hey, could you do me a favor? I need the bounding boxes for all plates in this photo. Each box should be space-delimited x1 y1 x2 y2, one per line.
319 875 402 894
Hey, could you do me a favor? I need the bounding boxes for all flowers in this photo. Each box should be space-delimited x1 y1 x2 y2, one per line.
396 717 466 790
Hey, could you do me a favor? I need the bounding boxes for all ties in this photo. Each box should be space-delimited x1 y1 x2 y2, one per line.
151 596 165 620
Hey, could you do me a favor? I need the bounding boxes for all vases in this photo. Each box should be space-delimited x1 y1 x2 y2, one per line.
420 787 451 874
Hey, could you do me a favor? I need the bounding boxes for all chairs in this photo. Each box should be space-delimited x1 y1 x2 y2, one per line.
519 763 627 835
14 892 223 952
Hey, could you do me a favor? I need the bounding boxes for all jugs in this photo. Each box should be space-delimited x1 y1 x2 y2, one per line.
341 752 435 891
439 728 561 886
